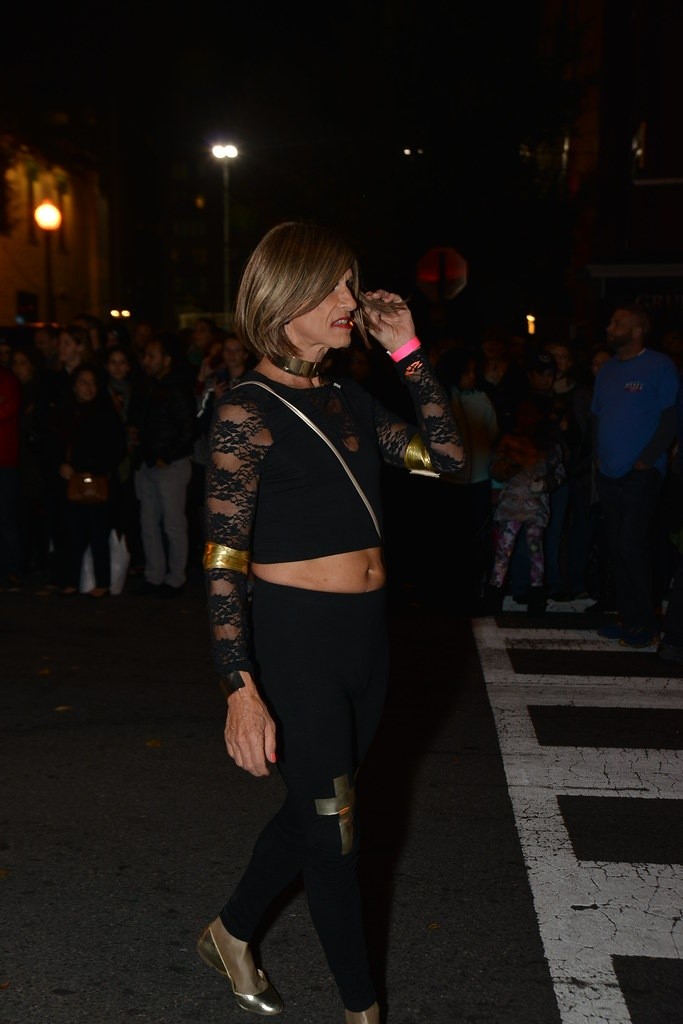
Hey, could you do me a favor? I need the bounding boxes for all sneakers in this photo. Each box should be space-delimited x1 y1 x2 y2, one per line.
599 620 627 638
620 626 662 647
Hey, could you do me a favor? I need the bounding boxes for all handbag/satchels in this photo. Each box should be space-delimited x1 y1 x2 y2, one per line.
65 473 110 505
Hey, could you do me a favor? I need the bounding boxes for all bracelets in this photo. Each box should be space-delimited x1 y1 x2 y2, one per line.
386 336 422 362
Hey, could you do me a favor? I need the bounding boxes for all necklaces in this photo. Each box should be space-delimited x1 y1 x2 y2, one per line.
266 351 321 379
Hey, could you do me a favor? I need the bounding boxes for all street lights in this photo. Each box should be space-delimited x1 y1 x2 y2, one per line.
35 204 62 334
206 138 243 335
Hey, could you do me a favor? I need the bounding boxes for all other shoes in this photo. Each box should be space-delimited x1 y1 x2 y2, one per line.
36 585 57 595
7 575 23 592
153 583 187 599
485 586 503 610
58 586 78 598
130 583 158 597
196 928 285 1015
512 585 546 604
84 587 108 599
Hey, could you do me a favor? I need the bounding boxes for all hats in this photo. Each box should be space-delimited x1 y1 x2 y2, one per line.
528 351 559 374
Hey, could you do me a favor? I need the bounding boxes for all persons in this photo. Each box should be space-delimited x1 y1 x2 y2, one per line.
198 219 472 1023
0 301 683 661
